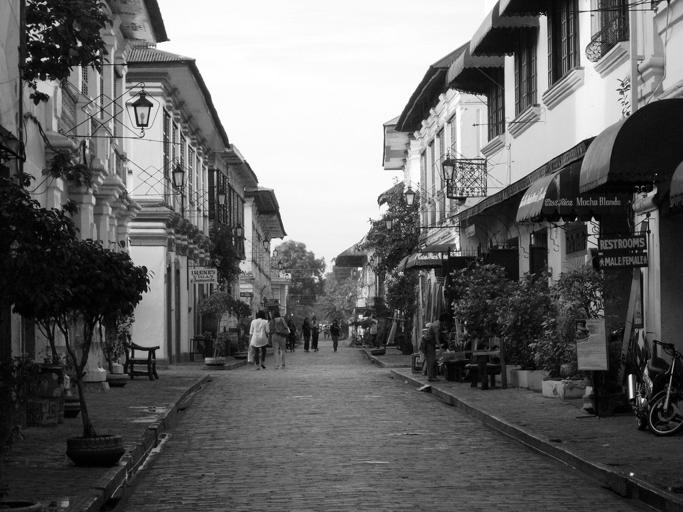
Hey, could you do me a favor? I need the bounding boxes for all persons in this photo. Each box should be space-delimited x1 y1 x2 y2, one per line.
287 318 297 352
310 315 320 352
420 313 452 382
248 310 269 371
330 319 341 352
302 317 314 352
268 311 292 369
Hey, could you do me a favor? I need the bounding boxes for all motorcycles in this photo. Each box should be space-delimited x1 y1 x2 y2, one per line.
628 339 681 436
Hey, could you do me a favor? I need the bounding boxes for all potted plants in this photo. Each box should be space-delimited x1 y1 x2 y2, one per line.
450 262 621 397
347 297 393 354
1 165 152 467
198 292 253 365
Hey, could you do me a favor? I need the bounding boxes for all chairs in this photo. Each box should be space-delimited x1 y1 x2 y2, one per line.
123 328 160 381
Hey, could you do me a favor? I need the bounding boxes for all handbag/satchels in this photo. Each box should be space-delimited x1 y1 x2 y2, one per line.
248 345 257 365
275 318 291 337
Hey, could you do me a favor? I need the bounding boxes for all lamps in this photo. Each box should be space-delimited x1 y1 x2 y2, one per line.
59 79 161 139
191 187 242 238
125 156 184 196
444 147 510 198
382 177 457 231
